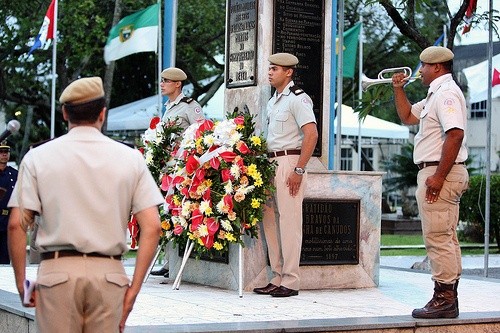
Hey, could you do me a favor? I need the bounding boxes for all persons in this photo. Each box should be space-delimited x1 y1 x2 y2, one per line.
5 75 166 333
147 68 202 278
0 142 45 266
252 52 318 296
390 46 471 318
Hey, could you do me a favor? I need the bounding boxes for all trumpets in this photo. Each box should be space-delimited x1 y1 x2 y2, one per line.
360 67 423 92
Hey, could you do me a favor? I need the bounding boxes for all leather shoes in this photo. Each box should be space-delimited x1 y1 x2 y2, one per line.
151 268 168 275
164 272 169 277
270 286 298 296
254 283 278 294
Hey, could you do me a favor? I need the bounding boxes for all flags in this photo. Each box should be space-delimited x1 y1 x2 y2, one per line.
25 0 56 61
104 0 161 64
335 0 500 106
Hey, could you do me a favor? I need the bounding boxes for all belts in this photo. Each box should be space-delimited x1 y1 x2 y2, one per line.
40 249 122 260
267 150 301 158
416 162 465 171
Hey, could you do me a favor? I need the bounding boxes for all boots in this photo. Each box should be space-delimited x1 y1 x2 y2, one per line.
412 281 459 318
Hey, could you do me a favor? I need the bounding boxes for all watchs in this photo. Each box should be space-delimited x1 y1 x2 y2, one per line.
294 166 306 175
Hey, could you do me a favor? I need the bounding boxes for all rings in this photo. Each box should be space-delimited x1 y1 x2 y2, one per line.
433 192 435 195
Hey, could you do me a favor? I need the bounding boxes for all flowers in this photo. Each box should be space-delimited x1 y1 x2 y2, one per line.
129 104 279 262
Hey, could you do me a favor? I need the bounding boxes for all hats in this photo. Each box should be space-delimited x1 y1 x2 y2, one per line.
269 53 299 68
0 140 15 152
160 68 187 81
420 46 454 63
59 76 104 104
